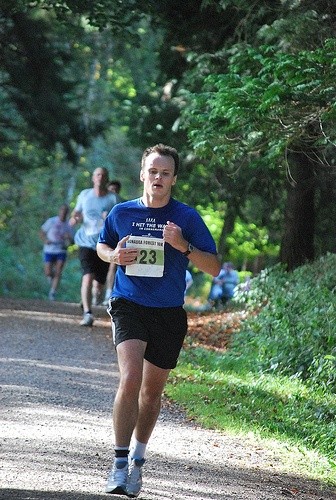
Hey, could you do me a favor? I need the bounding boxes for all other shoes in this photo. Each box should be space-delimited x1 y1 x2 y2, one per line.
91 288 102 305
80 313 93 326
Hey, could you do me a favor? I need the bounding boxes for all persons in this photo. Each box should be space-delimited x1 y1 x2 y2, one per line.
185 269 193 295
91 180 126 305
216 262 239 302
68 167 121 327
95 145 221 499
38 204 69 301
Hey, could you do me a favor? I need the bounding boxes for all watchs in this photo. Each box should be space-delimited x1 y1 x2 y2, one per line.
183 243 194 257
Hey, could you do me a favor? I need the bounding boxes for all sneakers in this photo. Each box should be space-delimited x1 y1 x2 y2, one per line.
126 457 146 498
106 458 129 495
